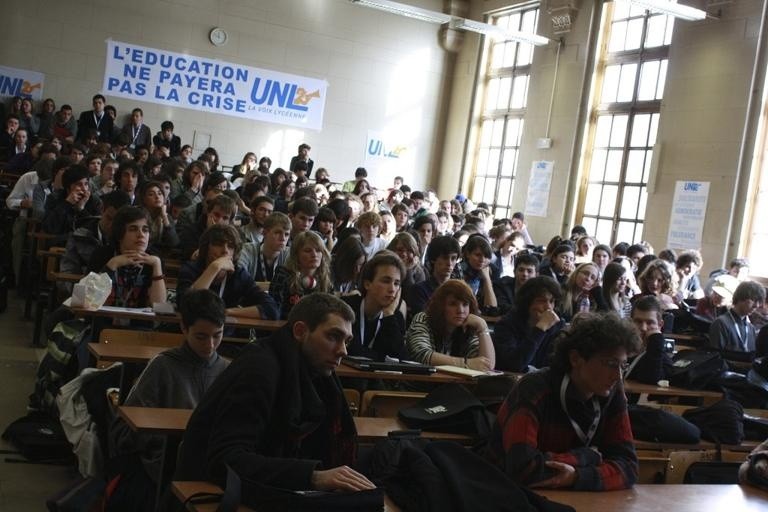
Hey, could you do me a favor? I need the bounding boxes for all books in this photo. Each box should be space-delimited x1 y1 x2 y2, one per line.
98 302 162 320
433 364 487 377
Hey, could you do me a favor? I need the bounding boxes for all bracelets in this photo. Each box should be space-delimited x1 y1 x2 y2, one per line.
149 273 168 281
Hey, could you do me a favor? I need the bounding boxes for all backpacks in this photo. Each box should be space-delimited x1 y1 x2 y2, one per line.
394 376 497 437
627 398 704 450
678 389 748 457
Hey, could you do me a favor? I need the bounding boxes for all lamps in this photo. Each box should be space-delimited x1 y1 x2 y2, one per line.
353 1 550 44
641 1 705 24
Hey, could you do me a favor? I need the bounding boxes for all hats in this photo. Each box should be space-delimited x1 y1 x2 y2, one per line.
712 275 740 298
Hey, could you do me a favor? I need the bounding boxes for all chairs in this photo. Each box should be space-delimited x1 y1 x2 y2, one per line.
665 452 748 485
363 388 432 419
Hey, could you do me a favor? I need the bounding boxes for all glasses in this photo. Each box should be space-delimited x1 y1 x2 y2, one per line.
582 354 629 370
578 269 598 280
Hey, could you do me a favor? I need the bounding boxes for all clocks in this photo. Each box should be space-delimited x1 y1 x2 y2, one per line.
210 28 228 47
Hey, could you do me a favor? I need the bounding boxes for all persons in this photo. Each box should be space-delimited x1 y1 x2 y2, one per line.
176 224 280 320
706 281 767 372
348 251 406 360
178 292 378 494
492 254 540 312
494 273 568 375
60 189 132 293
496 313 647 490
705 259 750 298
746 369 768 392
622 295 673 386
0 92 533 251
331 236 364 298
539 228 676 261
671 248 705 300
402 233 464 328
448 232 501 318
601 262 632 319
236 212 292 282
695 286 727 321
539 244 575 281
564 262 609 320
629 259 678 311
404 278 497 372
100 205 167 308
268 230 334 312
123 289 235 409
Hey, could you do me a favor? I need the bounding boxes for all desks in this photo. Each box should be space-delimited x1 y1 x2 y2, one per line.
529 484 768 512
66 306 738 356
120 415 768 453
32 270 96 347
89 330 768 395
